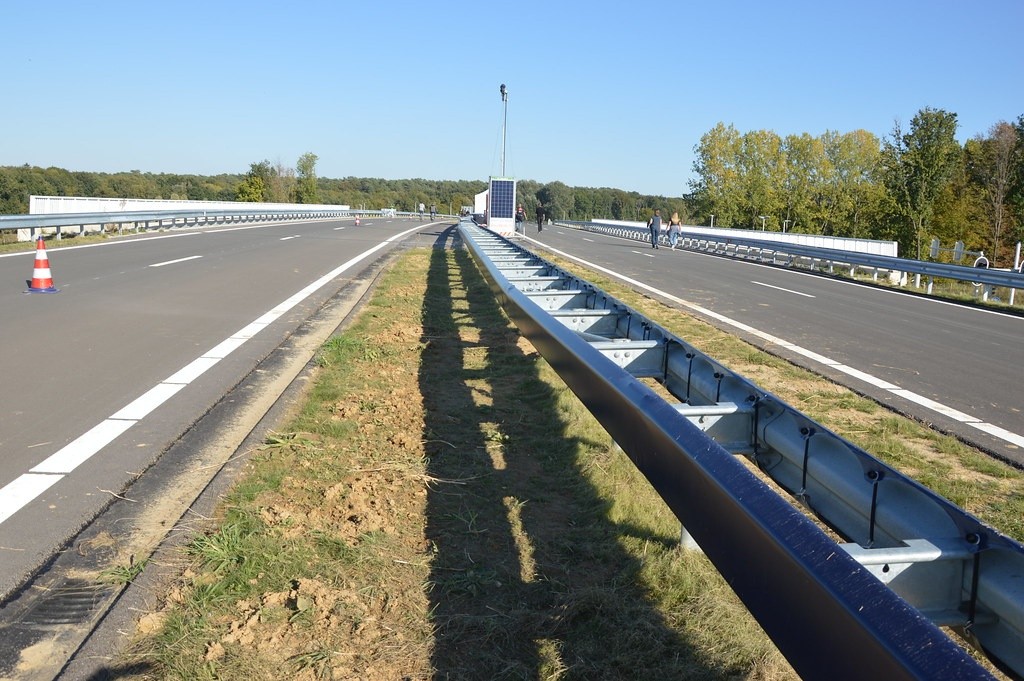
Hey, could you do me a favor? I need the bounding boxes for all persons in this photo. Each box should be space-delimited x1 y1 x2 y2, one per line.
419 201 425 217
515 204 527 233
535 203 545 233
430 204 438 219
647 209 662 249
666 210 682 251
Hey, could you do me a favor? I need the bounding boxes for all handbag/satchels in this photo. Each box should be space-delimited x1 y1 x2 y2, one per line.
543 213 545 220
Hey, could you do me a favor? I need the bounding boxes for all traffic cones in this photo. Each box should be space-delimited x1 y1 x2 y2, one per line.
408 213 414 222
354 213 362 227
25 235 58 293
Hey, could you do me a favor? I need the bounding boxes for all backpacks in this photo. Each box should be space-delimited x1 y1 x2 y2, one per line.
517 209 524 222
